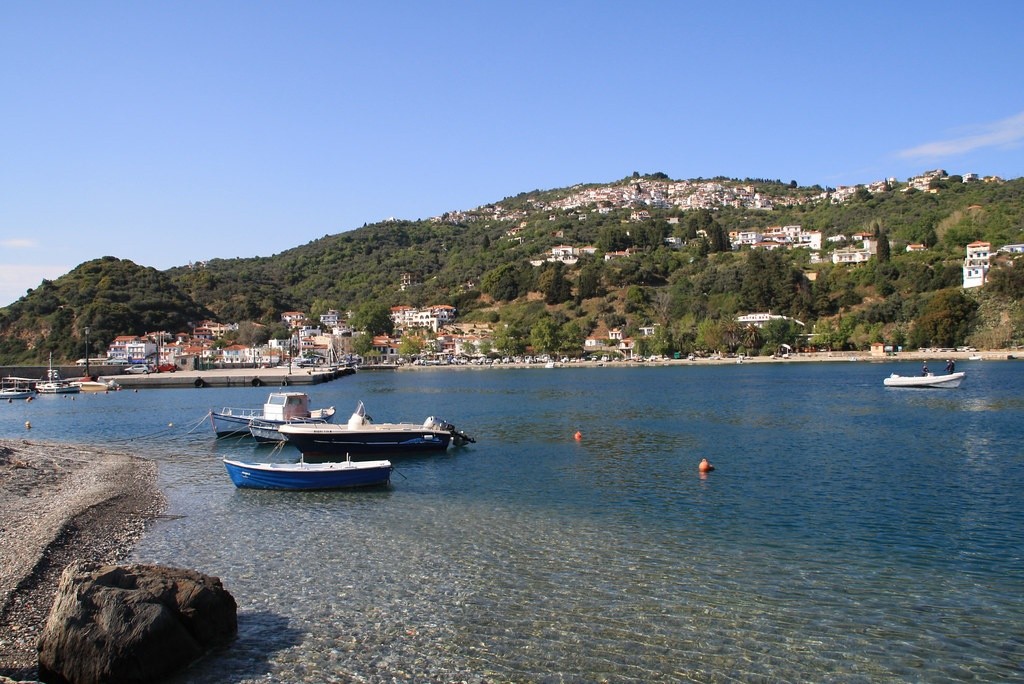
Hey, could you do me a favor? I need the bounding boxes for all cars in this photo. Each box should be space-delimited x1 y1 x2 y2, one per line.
296 353 672 368
154 362 177 373
124 363 153 374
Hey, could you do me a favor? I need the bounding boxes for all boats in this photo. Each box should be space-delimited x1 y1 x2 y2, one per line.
208 406 337 440
0 377 42 399
36 351 81 393
277 399 478 462
247 401 374 444
883 373 966 390
220 457 392 490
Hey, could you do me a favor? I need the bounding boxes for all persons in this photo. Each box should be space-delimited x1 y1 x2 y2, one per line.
943 360 955 375
922 365 929 376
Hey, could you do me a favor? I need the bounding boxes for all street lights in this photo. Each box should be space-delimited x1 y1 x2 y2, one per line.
83 327 91 377
